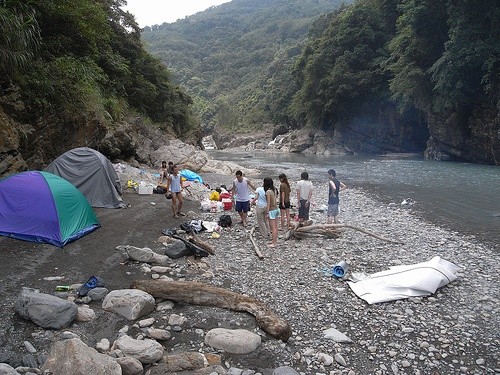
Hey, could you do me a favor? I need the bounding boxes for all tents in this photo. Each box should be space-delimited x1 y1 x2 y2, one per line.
0 169 103 248
42 145 127 210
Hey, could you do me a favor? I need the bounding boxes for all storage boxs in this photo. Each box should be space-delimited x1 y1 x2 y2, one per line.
222 198 232 210
139 184 154 196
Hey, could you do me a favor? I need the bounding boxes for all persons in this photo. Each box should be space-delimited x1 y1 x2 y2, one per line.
252 185 270 239
167 161 175 182
230 170 257 227
159 160 167 184
278 173 291 231
263 177 279 247
296 171 313 227
327 168 347 224
167 164 186 219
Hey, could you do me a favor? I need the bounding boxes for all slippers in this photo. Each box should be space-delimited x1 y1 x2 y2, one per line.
173 214 179 219
178 213 186 216
